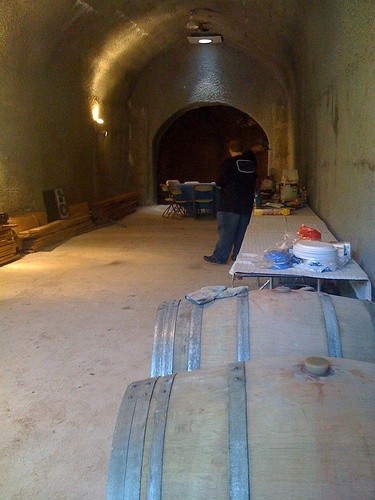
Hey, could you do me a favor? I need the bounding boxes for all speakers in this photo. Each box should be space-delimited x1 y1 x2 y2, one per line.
43 187 69 222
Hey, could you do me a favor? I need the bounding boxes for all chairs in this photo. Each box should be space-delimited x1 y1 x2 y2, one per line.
160 179 218 220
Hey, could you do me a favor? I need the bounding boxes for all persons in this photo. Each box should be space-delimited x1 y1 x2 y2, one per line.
242 137 270 167
204 136 256 266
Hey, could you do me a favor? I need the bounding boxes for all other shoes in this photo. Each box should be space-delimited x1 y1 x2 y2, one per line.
231 254 236 261
204 255 227 264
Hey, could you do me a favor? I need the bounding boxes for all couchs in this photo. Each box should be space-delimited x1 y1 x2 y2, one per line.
8 200 96 251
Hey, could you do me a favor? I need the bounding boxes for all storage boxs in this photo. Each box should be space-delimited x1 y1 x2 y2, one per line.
253 205 296 215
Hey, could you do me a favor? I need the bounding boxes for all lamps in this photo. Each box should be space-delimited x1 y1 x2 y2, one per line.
186 10 200 30
96 130 108 135
186 34 223 44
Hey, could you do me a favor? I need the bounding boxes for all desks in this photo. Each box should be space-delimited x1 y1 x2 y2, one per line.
228 197 372 302
172 183 222 216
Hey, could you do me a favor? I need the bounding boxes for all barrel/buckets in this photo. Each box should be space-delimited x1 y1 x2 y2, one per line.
151 286 375 378
104 356 375 500
